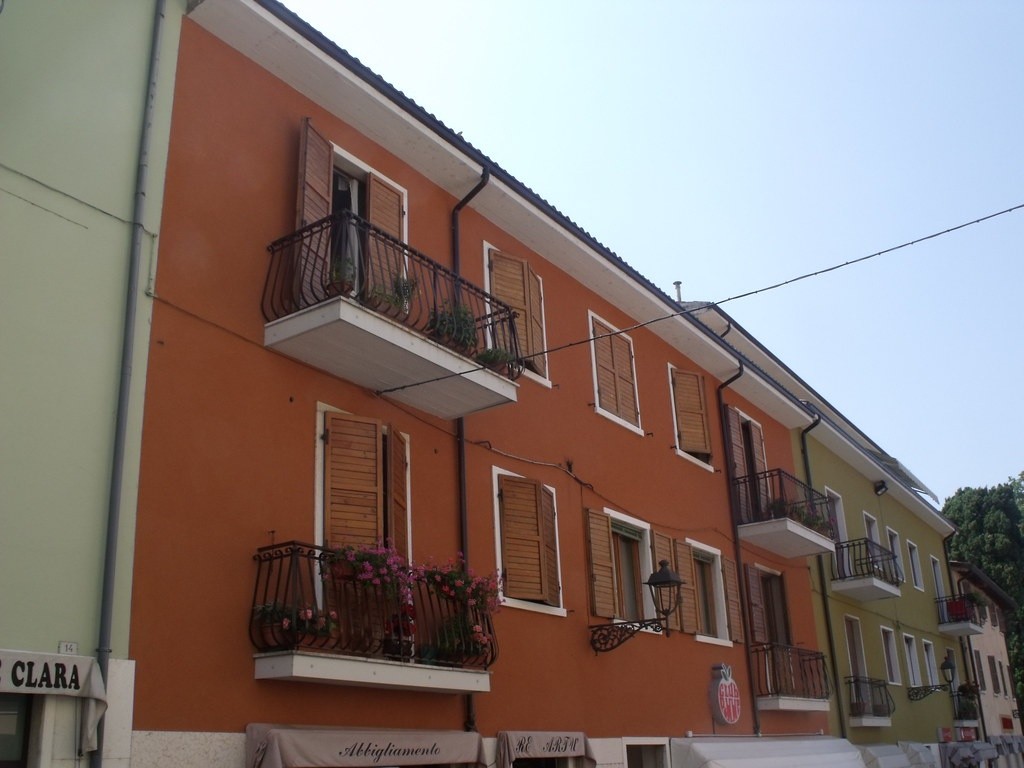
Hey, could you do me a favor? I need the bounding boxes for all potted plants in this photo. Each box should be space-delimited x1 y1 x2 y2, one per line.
328 257 357 297
431 302 515 378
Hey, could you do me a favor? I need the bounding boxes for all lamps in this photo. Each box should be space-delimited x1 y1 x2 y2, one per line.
942 656 956 684
646 560 684 636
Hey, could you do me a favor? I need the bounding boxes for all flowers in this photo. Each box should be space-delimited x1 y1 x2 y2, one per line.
277 542 502 664
